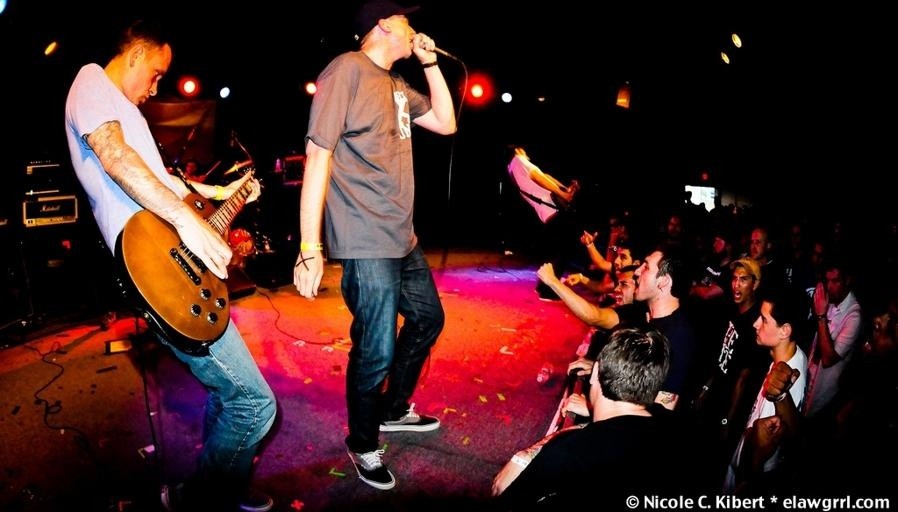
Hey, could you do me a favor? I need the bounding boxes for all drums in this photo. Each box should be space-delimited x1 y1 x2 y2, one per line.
224 158 256 184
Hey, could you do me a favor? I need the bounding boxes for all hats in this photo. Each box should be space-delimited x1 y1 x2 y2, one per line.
353 0 420 41
731 256 761 278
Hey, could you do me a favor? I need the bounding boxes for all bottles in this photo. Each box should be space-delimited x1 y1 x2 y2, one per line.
536 363 553 387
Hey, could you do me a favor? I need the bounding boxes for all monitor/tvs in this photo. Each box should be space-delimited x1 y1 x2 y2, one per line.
684 183 718 213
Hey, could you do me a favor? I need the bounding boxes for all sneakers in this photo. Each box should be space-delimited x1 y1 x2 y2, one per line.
379 403 440 433
240 484 274 511
536 283 560 300
160 480 183 512
347 447 396 489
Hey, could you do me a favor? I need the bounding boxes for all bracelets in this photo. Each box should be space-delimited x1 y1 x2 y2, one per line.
422 60 438 69
298 242 324 252
210 184 225 202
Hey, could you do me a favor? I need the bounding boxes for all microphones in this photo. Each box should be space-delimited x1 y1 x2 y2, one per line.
412 36 455 64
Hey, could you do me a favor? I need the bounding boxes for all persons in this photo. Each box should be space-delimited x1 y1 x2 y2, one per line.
62 19 277 510
291 0 458 492
486 137 894 506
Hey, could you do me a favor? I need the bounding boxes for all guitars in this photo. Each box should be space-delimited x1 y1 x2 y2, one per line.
550 179 580 213
113 167 266 353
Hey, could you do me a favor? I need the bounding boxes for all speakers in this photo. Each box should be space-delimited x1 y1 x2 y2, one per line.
16 194 81 318
